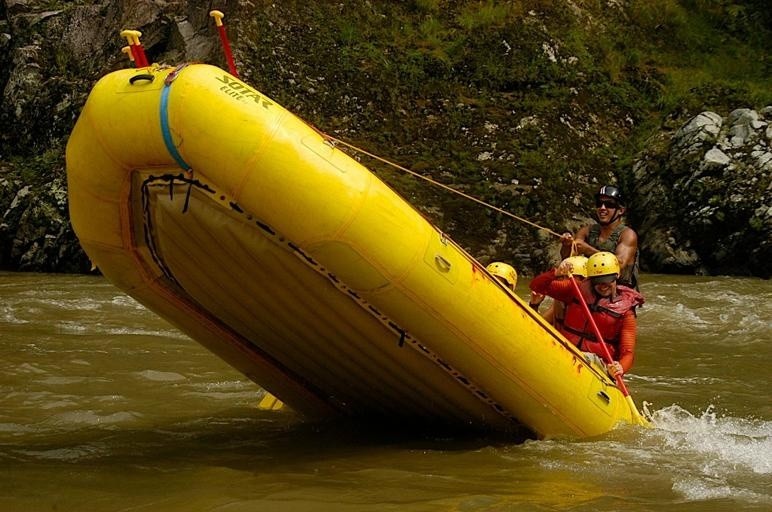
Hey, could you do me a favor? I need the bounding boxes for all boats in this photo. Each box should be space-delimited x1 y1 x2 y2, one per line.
61 61 661 447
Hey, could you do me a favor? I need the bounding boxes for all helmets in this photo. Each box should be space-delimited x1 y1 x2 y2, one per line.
558 254 588 279
588 250 622 279
595 185 627 205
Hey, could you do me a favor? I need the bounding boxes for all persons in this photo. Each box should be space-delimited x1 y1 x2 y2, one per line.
529 256 588 331
529 251 644 380
560 185 640 289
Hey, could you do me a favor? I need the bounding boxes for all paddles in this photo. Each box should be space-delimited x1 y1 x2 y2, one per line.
565 267 652 431
259 392 283 410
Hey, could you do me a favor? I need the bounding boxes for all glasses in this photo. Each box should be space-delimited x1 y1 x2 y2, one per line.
595 200 619 209
